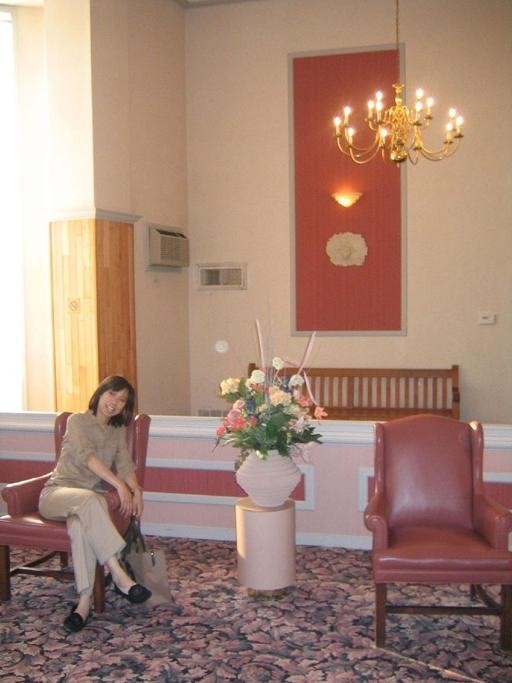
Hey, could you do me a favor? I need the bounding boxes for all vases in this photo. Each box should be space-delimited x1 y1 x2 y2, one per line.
235 450 303 507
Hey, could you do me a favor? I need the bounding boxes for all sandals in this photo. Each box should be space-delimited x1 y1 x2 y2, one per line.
63 604 89 631
113 582 153 604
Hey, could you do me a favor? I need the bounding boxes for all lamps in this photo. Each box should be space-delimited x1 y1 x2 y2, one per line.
331 191 362 208
333 0 465 167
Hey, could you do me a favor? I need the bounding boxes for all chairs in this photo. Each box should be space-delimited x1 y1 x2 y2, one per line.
1 412 151 614
363 414 512 647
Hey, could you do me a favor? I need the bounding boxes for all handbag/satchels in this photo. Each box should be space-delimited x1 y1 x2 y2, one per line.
119 516 175 609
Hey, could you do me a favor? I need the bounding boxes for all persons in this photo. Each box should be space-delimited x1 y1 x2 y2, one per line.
38 376 152 632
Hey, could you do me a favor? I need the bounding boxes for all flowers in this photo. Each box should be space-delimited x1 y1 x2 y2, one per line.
212 319 327 459
